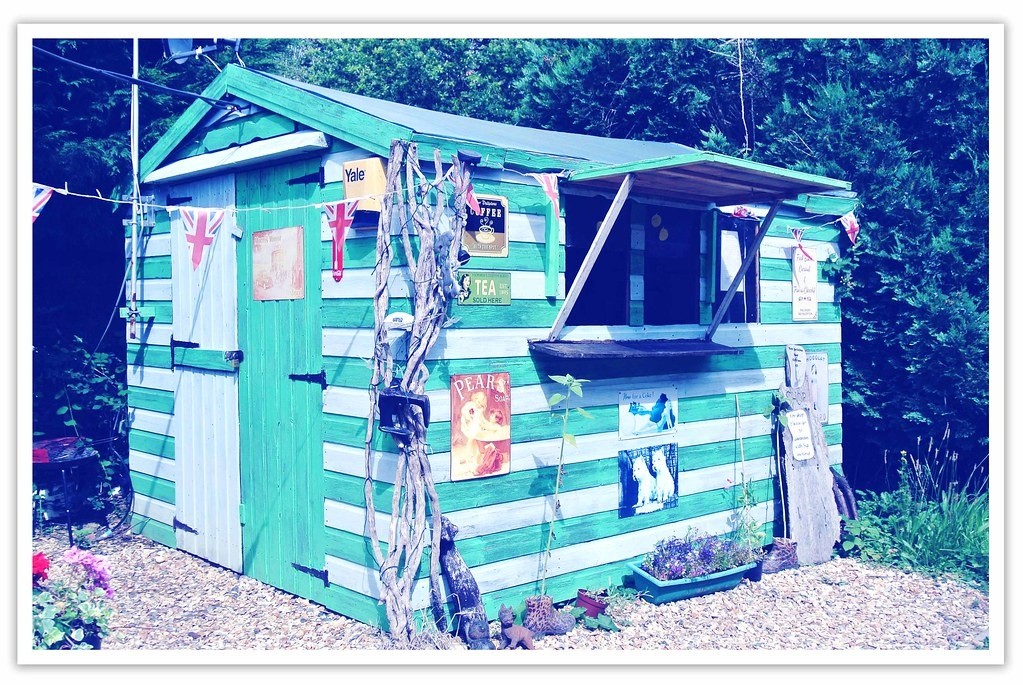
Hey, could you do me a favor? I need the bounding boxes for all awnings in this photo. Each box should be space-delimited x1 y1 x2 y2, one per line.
567 150 854 208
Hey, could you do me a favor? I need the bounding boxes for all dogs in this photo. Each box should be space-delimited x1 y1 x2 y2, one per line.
633 446 674 507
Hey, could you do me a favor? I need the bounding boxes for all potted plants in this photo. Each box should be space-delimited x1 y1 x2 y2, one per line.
518 368 650 631
763 392 799 571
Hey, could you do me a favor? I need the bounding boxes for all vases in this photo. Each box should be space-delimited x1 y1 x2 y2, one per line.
627 562 757 604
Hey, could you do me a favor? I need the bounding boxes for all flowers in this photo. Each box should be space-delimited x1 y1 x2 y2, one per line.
642 526 751 581
